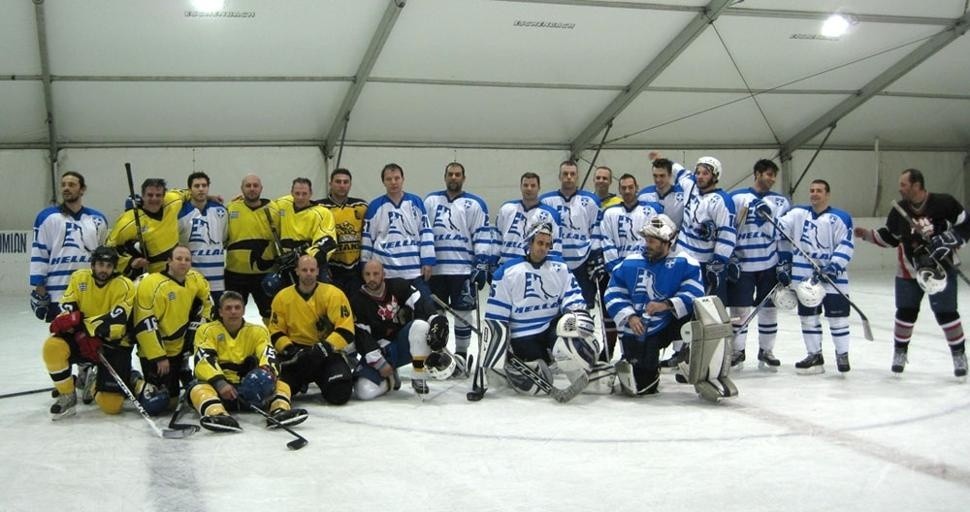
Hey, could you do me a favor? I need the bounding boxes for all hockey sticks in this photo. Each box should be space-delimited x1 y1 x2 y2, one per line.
250 403 308 450
595 278 617 396
760 210 873 341
95 349 200 439
430 282 589 403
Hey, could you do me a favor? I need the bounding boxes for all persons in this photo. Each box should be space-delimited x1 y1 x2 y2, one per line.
854 155 970 377
29 160 491 432
484 155 855 394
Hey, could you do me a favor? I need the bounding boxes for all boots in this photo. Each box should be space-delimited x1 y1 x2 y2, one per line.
658 350 689 367
834 349 850 372
731 349 746 367
893 346 908 373
757 347 781 366
796 350 824 369
950 349 967 376
411 376 429 394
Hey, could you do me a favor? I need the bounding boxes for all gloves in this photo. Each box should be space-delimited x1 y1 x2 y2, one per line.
29 287 54 321
48 310 81 334
392 304 415 327
274 241 310 275
725 261 742 284
775 263 793 287
704 264 722 289
74 331 103 364
816 261 843 283
587 256 604 283
927 228 965 261
469 262 487 295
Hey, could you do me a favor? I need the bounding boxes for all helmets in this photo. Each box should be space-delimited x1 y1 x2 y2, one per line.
421 350 456 381
915 264 948 296
125 194 143 210
694 156 721 180
263 273 282 296
240 364 277 403
552 334 601 375
636 214 679 247
772 287 796 311
795 281 826 308
91 244 119 263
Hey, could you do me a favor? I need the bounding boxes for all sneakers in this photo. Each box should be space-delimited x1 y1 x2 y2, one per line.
128 370 144 397
266 408 309 431
51 393 79 415
81 370 98 406
199 413 239 432
